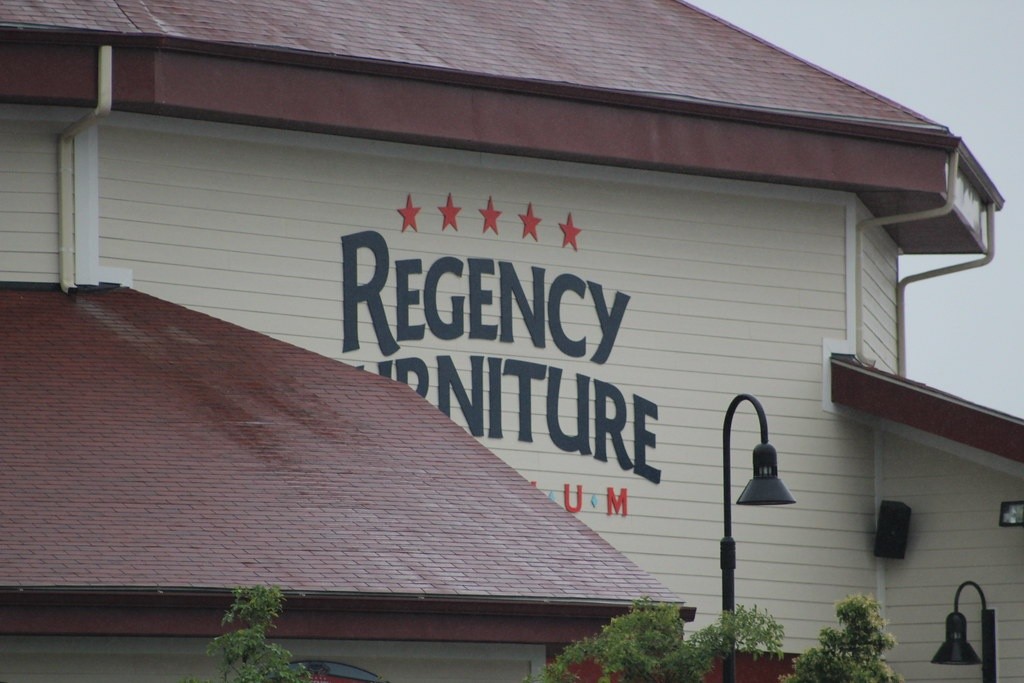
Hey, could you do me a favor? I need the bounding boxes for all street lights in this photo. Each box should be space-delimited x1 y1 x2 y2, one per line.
715 390 795 683
929 581 1000 683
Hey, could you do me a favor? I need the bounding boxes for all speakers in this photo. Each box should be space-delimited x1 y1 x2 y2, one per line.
874 500 911 559
